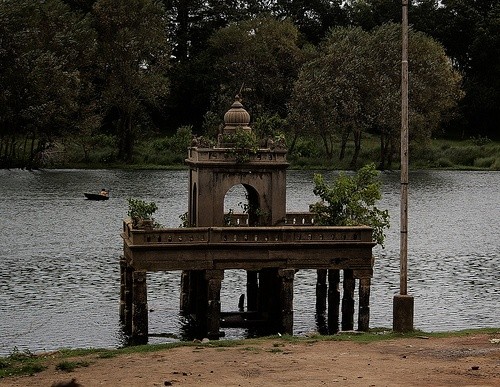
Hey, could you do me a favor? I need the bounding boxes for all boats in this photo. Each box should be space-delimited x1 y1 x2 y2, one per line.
84 192 109 201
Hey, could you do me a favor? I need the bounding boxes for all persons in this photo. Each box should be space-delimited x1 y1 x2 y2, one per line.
237 293 245 312
100 188 108 196
